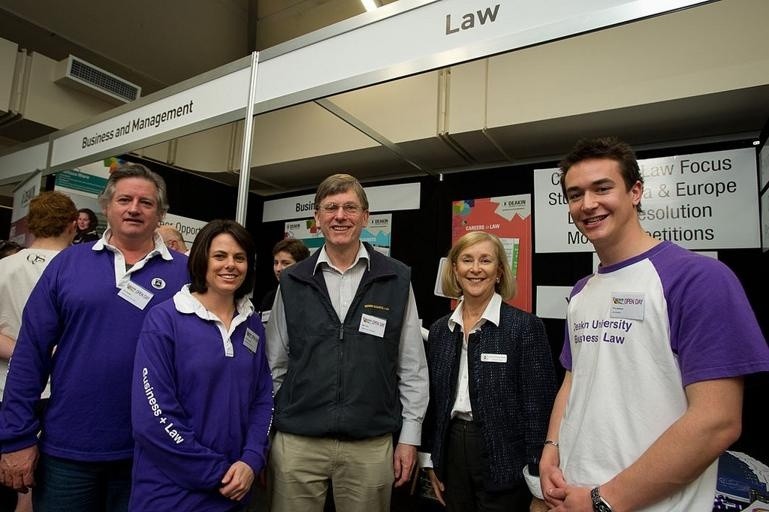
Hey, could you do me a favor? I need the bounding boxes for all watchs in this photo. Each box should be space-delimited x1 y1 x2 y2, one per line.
591 488 614 512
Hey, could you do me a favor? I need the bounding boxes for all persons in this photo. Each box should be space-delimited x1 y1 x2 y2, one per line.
265 174 430 512
72 209 99 244
256 238 311 331
530 136 768 512
0 191 81 512
127 220 276 512
154 226 191 257
409 230 560 512
0 162 189 512
0 242 22 261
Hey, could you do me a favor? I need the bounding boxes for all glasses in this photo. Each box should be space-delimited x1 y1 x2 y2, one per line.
321 203 363 214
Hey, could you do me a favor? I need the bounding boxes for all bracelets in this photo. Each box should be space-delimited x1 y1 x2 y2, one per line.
541 439 559 449
